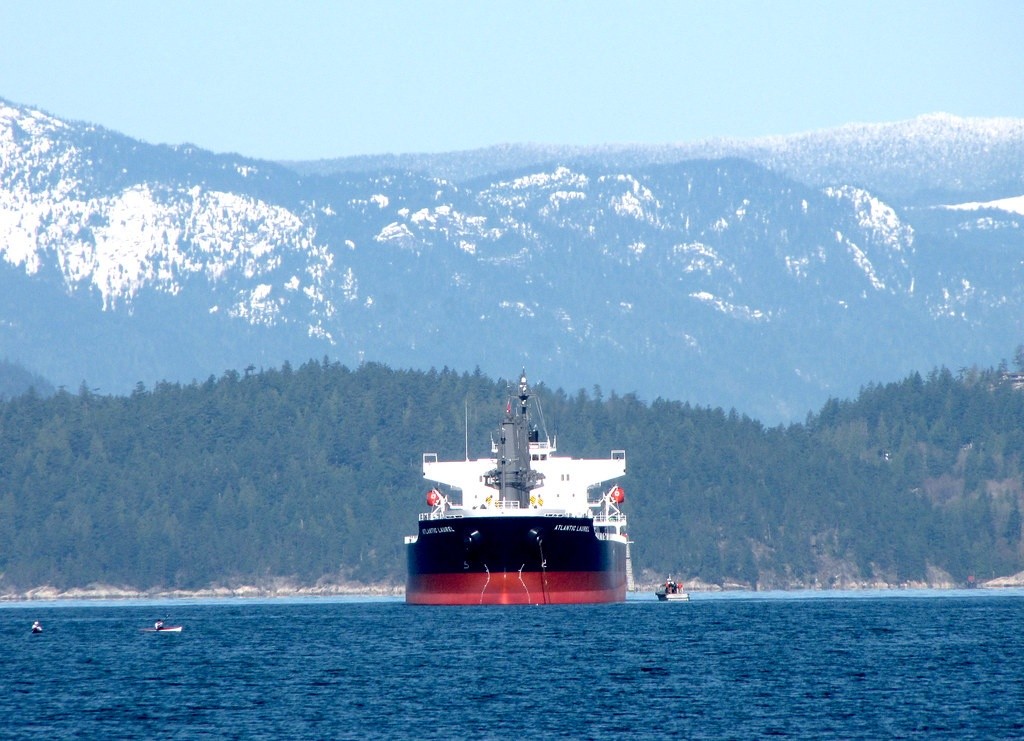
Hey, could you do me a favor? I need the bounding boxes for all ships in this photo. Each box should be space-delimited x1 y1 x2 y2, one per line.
402 367 629 606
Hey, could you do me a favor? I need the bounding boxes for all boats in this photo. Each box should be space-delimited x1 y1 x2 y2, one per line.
655 574 690 601
143 626 183 632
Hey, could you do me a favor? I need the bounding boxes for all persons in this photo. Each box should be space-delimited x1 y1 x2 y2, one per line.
665 580 683 594
